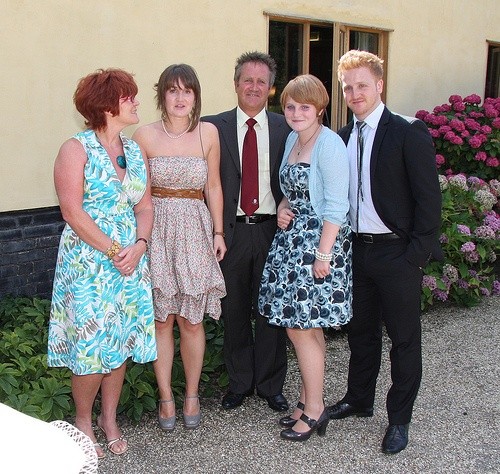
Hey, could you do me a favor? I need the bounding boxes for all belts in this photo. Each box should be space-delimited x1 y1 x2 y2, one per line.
236 214 276 225
350 232 400 243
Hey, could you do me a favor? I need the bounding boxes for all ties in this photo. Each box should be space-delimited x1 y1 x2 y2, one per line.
241 118 260 216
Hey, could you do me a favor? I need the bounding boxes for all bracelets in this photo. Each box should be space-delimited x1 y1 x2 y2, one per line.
314 249 333 262
135 238 148 244
103 240 121 260
213 232 226 238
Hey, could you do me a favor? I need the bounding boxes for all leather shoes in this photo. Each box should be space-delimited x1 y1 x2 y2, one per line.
381 424 409 454
222 387 254 409
257 391 289 412
325 399 373 419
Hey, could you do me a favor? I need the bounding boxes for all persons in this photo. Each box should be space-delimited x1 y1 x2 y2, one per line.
198 49 292 413
47 66 155 459
324 48 444 455
258 72 353 442
130 62 227 430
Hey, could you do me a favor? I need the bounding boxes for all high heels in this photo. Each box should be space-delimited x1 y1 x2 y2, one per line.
158 390 176 430
183 392 202 427
280 397 329 443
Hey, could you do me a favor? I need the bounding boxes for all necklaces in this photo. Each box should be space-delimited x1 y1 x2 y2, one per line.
297 124 320 156
101 144 126 169
161 119 193 139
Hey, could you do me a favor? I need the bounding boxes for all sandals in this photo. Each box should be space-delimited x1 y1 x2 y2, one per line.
97 417 128 455
73 423 106 458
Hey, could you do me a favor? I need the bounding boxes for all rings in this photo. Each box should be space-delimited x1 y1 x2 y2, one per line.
281 224 284 227
128 267 131 270
